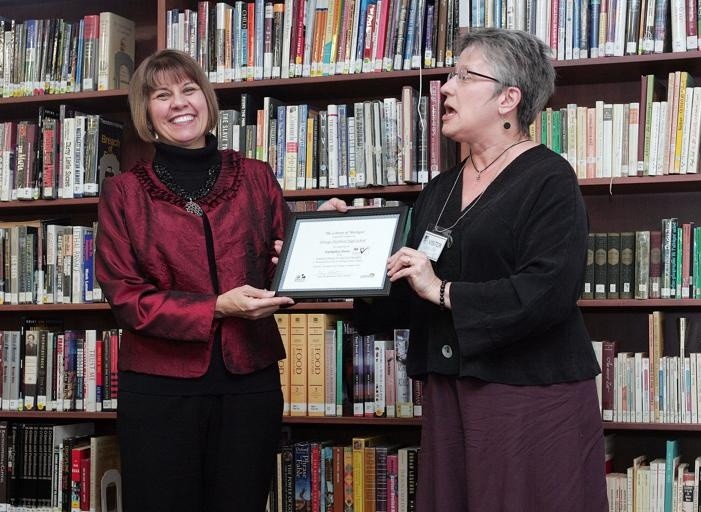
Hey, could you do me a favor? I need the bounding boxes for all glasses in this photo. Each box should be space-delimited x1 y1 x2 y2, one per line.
446 65 501 87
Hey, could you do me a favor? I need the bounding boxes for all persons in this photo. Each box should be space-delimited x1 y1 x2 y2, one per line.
331 27 608 512
96 49 347 512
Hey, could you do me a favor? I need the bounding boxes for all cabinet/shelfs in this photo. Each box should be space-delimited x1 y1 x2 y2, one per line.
0 0 700 512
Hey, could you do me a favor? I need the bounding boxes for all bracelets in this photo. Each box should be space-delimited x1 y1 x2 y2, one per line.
440 279 448 306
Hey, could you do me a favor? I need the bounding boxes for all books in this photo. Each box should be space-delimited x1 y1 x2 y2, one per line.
576 217 701 512
162 2 701 191
164 197 436 512
0 0 136 512
